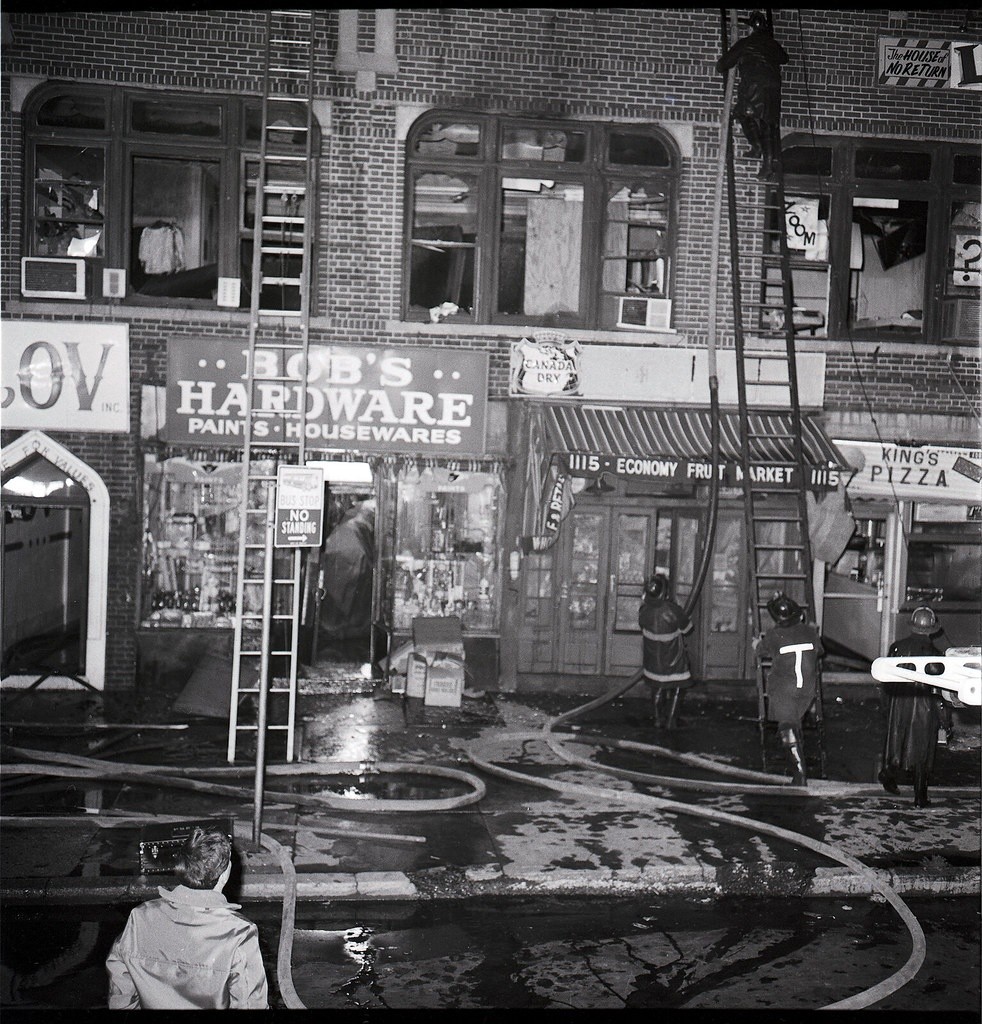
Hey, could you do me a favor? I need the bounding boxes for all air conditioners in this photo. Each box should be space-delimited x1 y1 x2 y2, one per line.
20 256 87 301
602 295 677 334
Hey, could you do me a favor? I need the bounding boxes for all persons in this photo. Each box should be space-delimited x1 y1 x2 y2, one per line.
716 10 789 180
639 573 694 729
752 593 826 788
878 603 954 807
106 824 268 1010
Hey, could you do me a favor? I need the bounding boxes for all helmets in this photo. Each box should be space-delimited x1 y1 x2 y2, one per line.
641 573 668 606
909 605 941 636
768 596 802 624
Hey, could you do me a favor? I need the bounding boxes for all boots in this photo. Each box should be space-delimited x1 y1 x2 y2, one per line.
780 744 808 786
664 687 686 734
879 758 900 795
650 684 666 729
913 769 931 809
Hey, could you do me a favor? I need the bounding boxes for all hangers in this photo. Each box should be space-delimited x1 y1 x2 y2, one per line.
146 219 174 230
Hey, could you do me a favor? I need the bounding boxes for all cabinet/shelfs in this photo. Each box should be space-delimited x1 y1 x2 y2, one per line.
29 139 108 264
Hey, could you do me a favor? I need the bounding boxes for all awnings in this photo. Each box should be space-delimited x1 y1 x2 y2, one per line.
544 404 851 491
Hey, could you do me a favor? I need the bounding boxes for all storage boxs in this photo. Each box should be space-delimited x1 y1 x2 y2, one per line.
405 653 426 698
413 617 466 658
426 651 464 694
424 668 466 707
379 639 414 676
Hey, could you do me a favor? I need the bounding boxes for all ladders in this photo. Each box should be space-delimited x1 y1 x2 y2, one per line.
222 11 319 762
706 5 833 777
